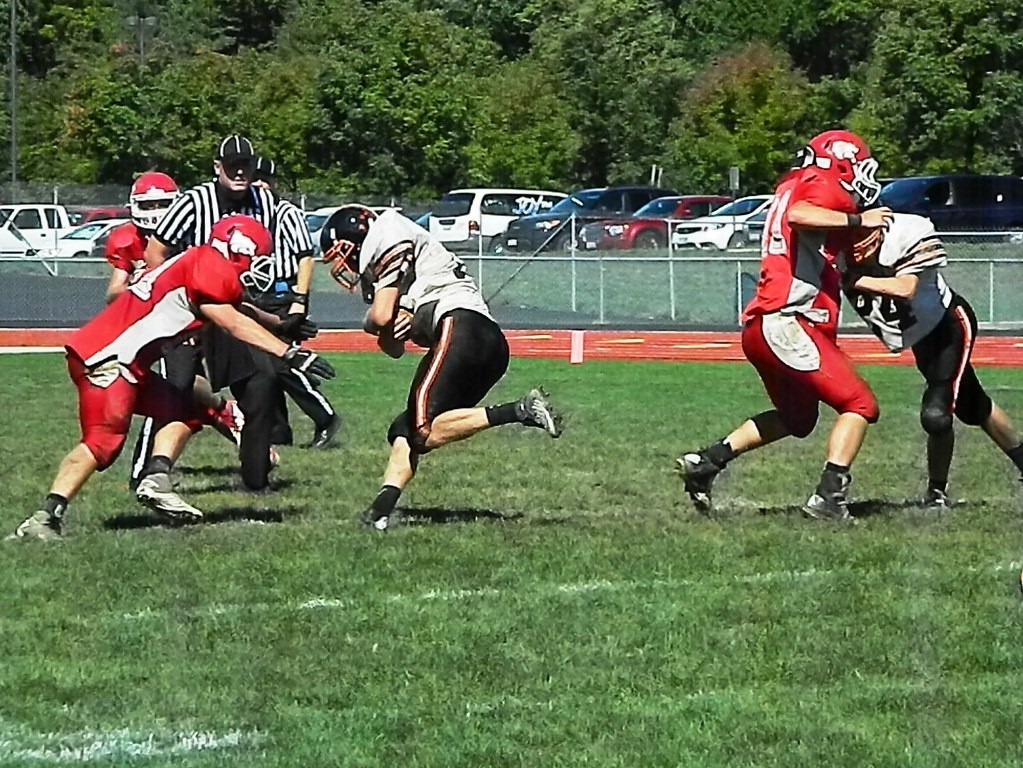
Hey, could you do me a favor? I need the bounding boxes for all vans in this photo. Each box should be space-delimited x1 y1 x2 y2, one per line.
305 206 403 258
65 207 132 226
429 189 569 254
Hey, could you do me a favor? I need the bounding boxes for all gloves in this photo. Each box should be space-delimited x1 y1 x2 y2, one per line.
839 266 859 288
378 334 403 358
361 309 380 337
283 285 309 335
281 316 318 341
282 342 336 386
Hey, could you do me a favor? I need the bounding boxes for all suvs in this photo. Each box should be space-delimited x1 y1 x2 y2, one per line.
578 195 736 252
503 186 679 258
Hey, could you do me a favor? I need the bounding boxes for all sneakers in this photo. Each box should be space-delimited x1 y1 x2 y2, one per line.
677 448 719 517
348 511 387 533
801 486 854 523
136 472 203 522
234 430 280 467
17 509 64 541
221 397 244 438
516 386 561 439
303 413 342 451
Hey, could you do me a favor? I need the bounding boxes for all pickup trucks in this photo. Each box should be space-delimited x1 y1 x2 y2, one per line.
0 204 84 258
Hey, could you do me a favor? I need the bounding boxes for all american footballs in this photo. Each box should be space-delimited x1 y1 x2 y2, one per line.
378 295 413 343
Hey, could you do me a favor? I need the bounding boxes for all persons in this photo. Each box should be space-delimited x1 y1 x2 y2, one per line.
320 205 566 529
15 215 336 540
128 134 315 489
836 198 1023 510
105 172 342 464
677 130 896 522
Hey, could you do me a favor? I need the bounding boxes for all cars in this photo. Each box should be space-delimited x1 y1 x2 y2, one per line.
414 211 433 231
671 194 775 252
33 219 134 258
878 174 1023 243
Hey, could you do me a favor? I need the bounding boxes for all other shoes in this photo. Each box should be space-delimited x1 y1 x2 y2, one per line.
903 495 948 511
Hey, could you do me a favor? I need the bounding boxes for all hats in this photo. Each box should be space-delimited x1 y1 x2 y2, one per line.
214 134 254 160
256 157 275 174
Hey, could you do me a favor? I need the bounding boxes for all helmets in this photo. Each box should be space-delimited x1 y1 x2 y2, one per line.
320 203 378 290
208 215 274 300
839 190 883 214
803 131 871 184
130 172 180 235
